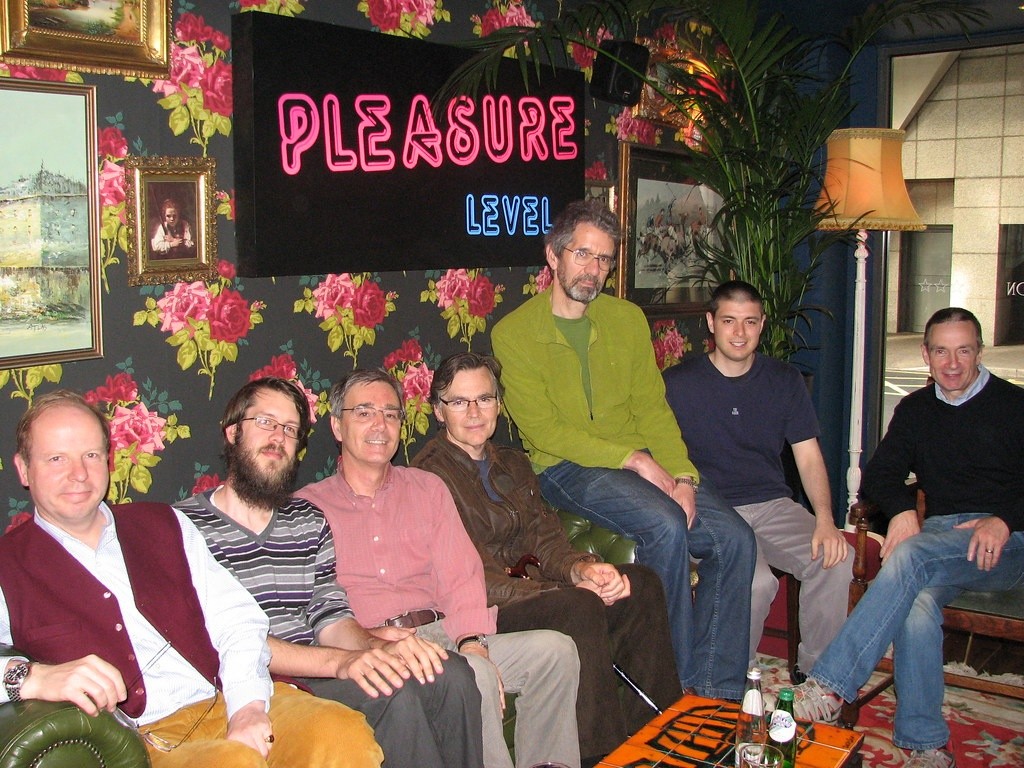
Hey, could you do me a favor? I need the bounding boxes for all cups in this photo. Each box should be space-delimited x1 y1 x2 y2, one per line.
740 744 784 768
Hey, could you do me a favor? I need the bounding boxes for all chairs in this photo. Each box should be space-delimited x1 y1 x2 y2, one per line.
843 485 1024 700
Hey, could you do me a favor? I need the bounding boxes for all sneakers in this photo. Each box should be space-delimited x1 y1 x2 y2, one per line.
900 739 955 768
760 675 843 725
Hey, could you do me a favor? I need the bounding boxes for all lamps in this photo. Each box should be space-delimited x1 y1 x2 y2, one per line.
811 126 929 529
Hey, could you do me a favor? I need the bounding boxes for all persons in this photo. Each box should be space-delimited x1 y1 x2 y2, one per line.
173 376 483 768
151 201 194 256
491 203 757 702
0 391 385 768
662 281 854 676
766 308 1024 768
291 371 584 768
412 354 682 768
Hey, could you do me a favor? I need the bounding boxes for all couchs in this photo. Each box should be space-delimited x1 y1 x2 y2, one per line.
0 511 637 768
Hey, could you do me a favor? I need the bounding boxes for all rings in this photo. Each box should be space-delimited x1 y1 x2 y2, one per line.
986 550 993 553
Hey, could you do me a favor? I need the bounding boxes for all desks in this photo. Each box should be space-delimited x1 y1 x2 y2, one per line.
594 689 867 767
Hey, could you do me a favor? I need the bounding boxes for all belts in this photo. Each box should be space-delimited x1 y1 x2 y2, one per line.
385 608 445 628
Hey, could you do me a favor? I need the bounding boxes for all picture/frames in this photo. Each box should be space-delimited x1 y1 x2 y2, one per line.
0 0 174 80
125 156 216 285
0 80 104 365
616 139 739 316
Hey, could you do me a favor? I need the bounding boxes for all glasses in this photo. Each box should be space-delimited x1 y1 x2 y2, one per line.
241 415 306 440
565 246 617 272
113 642 218 752
438 391 499 413
342 406 405 423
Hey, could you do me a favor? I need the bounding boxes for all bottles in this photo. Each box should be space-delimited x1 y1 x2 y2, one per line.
767 709 796 768
741 667 763 707
735 689 766 768
775 689 795 722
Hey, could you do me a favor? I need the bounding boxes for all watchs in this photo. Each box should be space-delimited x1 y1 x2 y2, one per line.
3 660 39 702
677 479 698 494
458 636 488 652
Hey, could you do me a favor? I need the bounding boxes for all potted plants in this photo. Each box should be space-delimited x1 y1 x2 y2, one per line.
411 0 988 494
